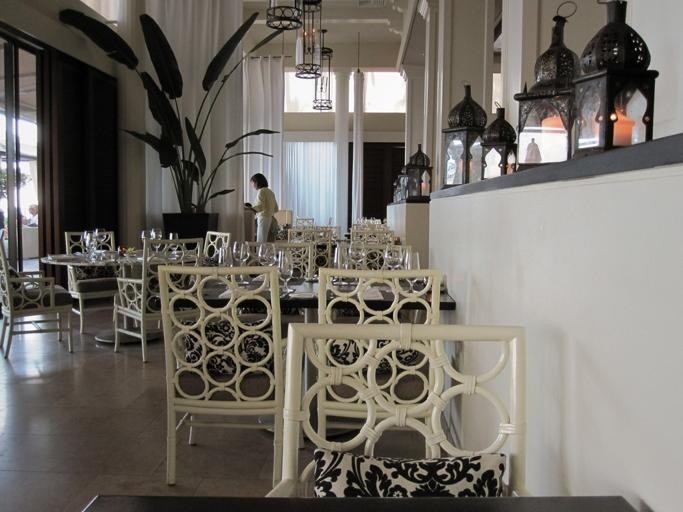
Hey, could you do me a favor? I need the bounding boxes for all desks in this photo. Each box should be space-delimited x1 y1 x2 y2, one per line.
169 280 456 440
268 246 404 274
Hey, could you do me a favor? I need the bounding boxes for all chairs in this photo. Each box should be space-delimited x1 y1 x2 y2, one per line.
288 229 331 279
316 226 341 241
303 269 441 458
113 238 204 362
157 231 230 329
350 231 394 244
331 245 410 324
158 265 306 489
65 232 128 334
296 218 314 229
352 223 388 230
0 229 73 359
339 243 407 273
237 242 314 349
267 323 527 497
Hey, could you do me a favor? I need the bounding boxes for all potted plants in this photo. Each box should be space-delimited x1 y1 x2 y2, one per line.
59 8 290 255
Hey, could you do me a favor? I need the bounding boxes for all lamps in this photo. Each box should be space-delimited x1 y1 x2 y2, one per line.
310 48 334 111
295 0 322 78
106 21 118 27
266 0 303 29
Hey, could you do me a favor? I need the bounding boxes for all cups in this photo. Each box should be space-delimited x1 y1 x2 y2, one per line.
404 250 420 295
277 250 294 294
384 244 404 271
347 240 366 269
150 227 163 257
217 247 233 286
84 227 107 264
169 232 180 260
141 229 152 245
257 242 277 291
232 240 252 286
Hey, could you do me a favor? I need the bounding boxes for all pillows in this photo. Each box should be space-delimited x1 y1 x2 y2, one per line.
147 274 197 298
333 310 408 318
204 256 217 267
328 340 419 373
8 265 26 294
238 306 298 314
73 265 116 279
180 319 286 376
314 450 507 497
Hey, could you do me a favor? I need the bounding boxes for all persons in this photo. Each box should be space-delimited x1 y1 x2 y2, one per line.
0 209 5 242
244 173 280 253
28 205 38 226
